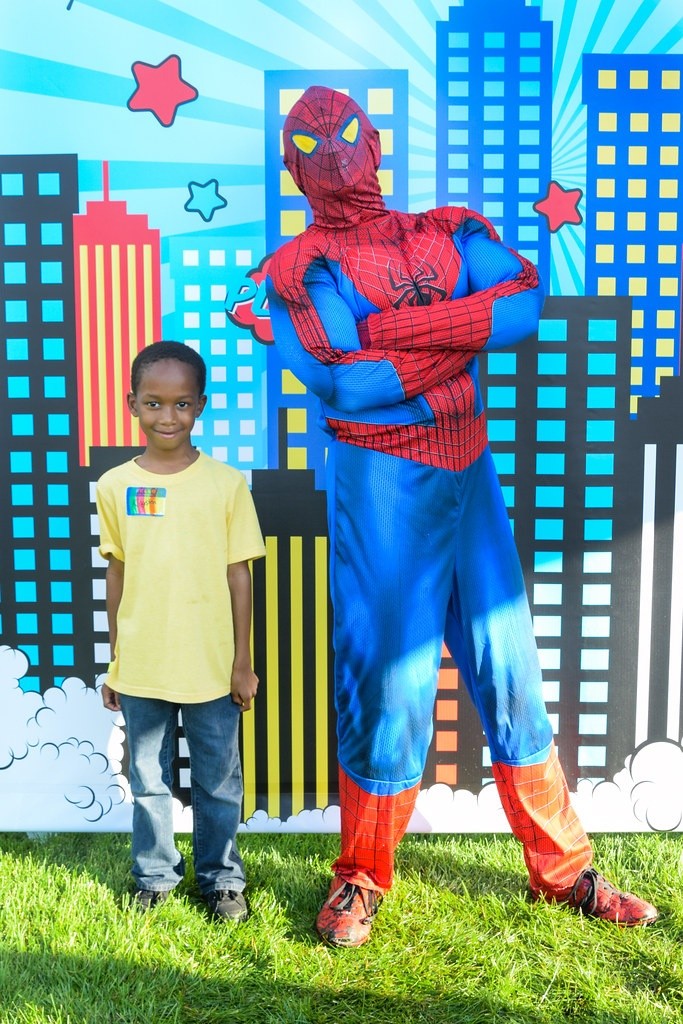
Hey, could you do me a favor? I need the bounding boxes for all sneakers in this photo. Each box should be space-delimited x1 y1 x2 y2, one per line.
316 876 383 947
530 863 658 925
132 887 169 911
206 889 248 921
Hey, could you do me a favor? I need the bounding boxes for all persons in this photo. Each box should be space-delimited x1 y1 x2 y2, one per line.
262 87 657 948
95 341 267 922
